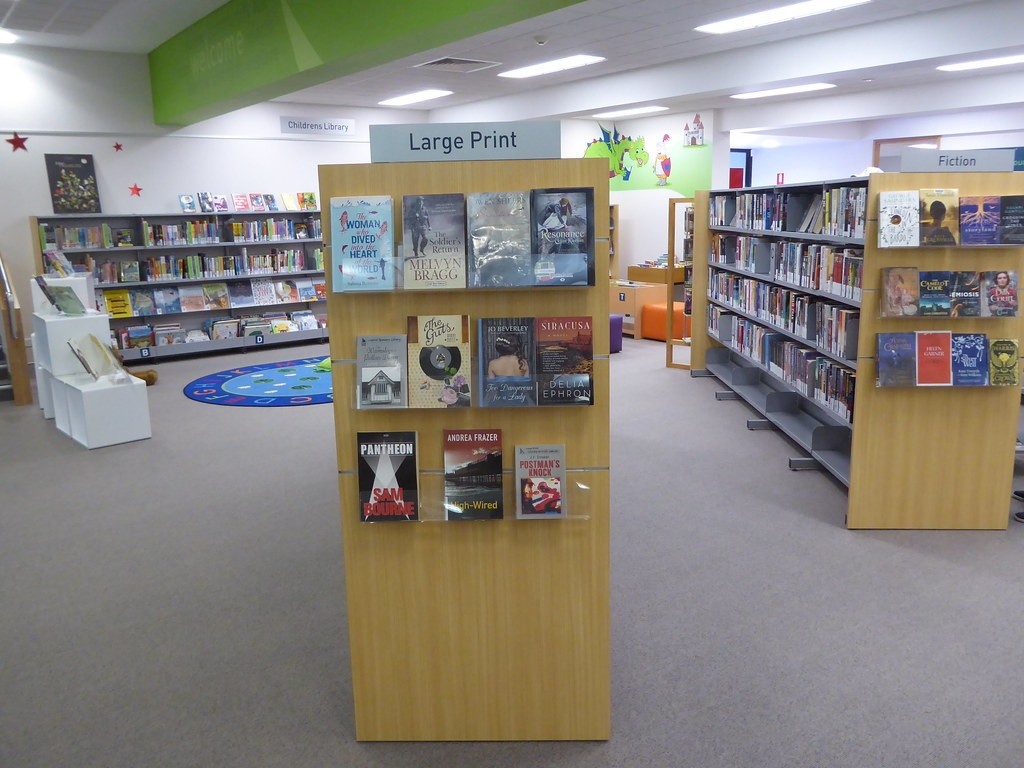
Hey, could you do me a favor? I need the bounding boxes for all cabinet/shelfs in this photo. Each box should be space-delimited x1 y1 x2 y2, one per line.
27 210 328 361
667 171 1024 531
319 157 612 743
610 204 619 280
30 271 153 450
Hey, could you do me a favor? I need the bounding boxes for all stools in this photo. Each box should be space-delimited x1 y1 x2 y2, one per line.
640 301 692 342
609 314 623 355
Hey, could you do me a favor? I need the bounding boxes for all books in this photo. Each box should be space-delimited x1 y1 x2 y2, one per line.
355 315 537 410
536 317 595 407
40 192 328 379
684 185 868 423
513 444 567 519
875 192 1023 390
357 431 420 522
442 427 504 519
633 251 685 269
330 187 596 290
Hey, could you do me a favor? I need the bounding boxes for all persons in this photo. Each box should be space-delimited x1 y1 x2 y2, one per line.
408 196 430 258
987 271 1017 318
52 165 98 212
521 478 562 513
537 196 569 232
925 200 956 245
488 331 530 379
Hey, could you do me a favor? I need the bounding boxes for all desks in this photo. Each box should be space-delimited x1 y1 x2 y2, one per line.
629 264 685 285
609 279 667 340
